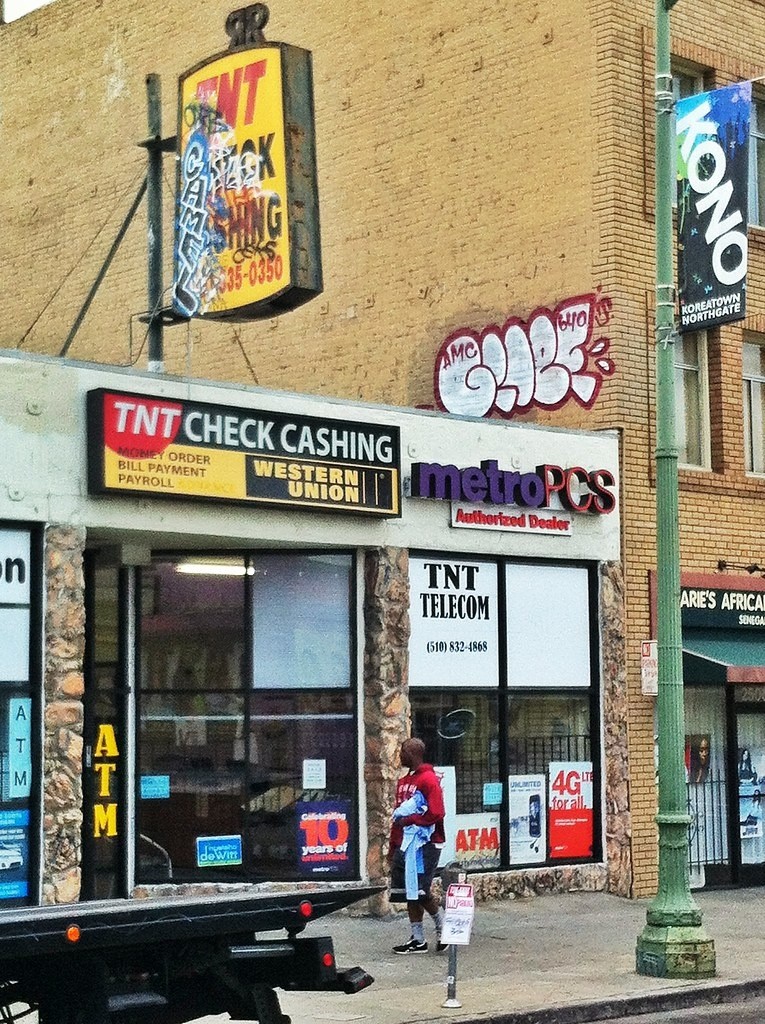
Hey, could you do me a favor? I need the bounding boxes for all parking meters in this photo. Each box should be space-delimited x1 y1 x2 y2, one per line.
441 862 470 1008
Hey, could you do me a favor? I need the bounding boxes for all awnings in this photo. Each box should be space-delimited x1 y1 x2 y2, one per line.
680 640 765 684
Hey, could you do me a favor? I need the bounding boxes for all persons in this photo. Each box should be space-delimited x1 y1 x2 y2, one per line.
384 738 450 955
688 734 711 782
737 791 760 826
738 750 757 785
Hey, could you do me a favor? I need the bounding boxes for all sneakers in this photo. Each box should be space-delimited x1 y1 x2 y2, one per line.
433 924 451 953
390 935 429 955
